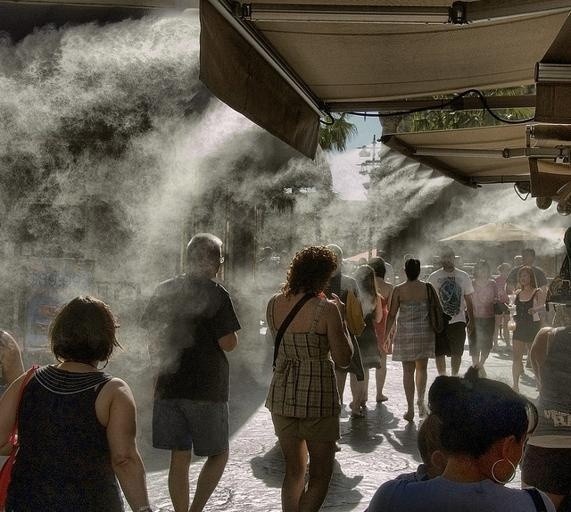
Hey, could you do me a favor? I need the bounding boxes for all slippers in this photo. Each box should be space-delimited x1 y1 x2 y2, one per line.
351 411 365 418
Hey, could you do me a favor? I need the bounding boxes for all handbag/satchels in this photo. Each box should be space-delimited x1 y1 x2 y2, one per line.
429 294 444 334
0 443 19 512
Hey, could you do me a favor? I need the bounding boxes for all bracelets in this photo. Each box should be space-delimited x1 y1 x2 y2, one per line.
138 506 150 512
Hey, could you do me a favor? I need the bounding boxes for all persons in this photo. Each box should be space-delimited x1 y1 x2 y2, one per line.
494 247 547 393
140 232 242 512
384 258 436 421
466 260 498 378
427 246 474 375
264 246 353 511
521 326 570 511
399 254 505 283
264 246 394 451
0 294 153 512
0 330 26 472
365 376 557 511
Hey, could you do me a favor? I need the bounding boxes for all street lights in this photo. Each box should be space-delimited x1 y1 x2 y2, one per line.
355 134 389 261
